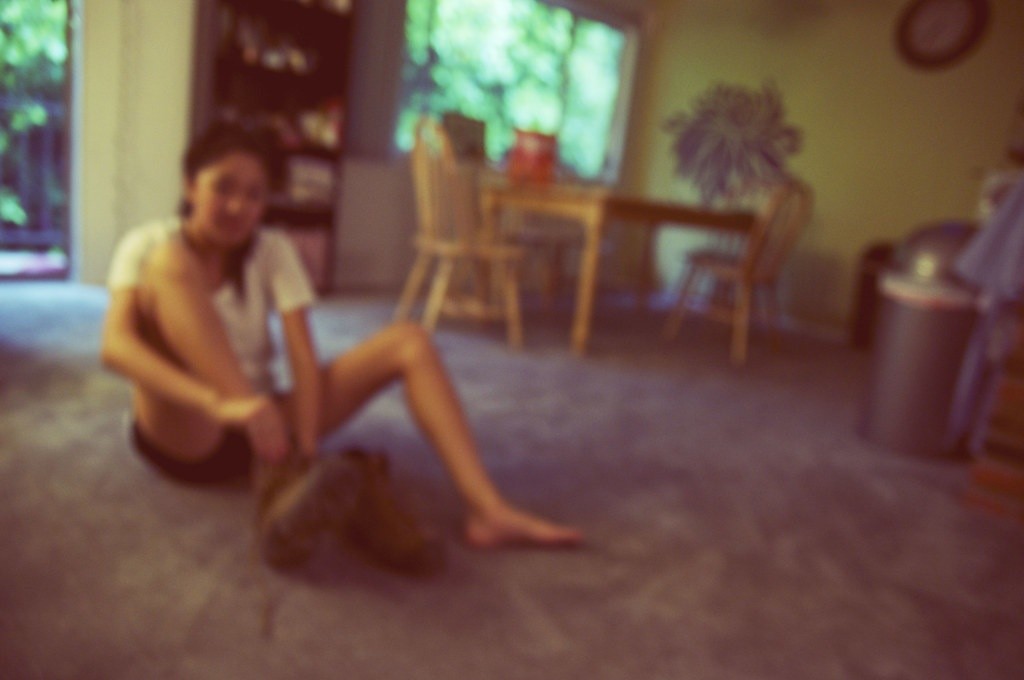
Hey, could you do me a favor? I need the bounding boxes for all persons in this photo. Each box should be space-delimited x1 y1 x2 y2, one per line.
102 123 584 564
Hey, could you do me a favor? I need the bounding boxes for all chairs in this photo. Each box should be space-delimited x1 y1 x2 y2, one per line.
395 113 812 346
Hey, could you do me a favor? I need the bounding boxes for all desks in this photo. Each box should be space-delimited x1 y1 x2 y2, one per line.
473 176 760 350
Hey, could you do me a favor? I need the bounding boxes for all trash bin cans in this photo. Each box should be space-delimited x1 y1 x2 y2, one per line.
852 219 994 460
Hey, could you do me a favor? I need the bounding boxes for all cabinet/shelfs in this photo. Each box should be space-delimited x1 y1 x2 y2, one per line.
189 0 358 297
